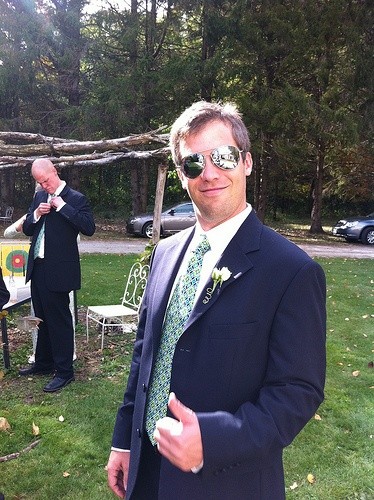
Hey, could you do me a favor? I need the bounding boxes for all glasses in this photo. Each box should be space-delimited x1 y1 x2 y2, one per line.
175 143 243 180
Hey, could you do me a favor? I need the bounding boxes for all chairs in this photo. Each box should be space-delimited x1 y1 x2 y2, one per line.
86 262 150 351
0 206 14 225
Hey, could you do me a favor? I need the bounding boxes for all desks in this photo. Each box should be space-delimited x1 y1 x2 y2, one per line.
0 289 79 368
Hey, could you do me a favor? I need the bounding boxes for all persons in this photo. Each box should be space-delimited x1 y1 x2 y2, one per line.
106 99 326 500
0 158 96 392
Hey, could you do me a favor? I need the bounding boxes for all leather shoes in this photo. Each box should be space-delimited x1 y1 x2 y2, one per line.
43 372 75 392
18 367 55 376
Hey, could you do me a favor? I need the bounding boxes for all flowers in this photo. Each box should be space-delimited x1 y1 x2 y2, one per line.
202 266 232 304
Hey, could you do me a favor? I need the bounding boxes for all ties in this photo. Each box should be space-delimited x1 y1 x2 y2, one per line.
143 232 211 448
33 192 56 261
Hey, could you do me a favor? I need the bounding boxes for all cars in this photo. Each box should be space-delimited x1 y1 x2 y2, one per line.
125 200 197 240
330 213 374 246
